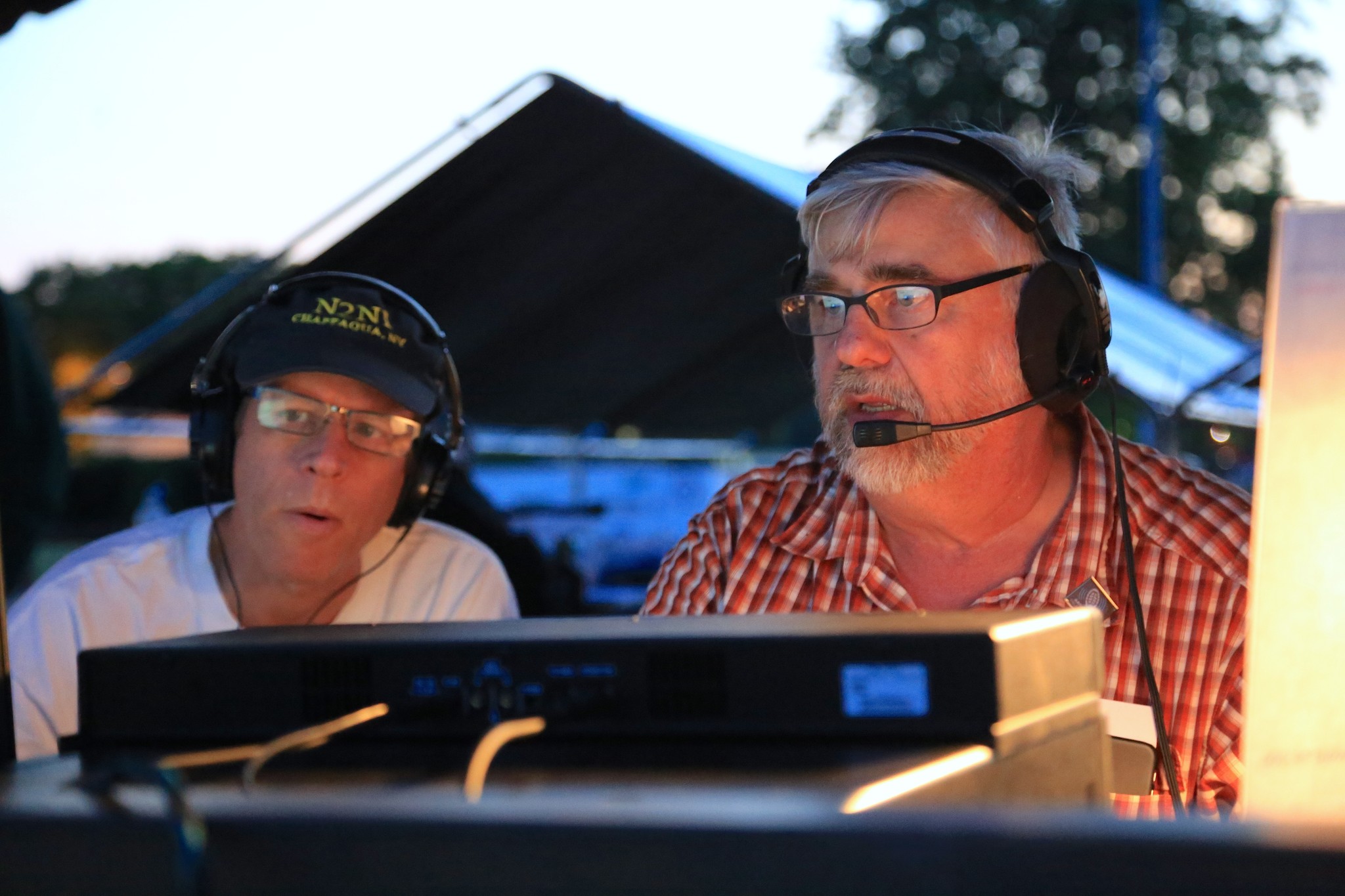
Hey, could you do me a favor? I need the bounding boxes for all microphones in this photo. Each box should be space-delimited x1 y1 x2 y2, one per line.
853 386 1072 448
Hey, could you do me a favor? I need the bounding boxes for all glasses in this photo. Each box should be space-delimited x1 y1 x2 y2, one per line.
240 382 423 459
777 263 1049 337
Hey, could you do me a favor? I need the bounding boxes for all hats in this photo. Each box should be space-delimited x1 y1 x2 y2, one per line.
228 276 448 417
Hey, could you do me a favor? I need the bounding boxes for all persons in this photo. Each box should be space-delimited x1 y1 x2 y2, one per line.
625 124 1256 829
4 264 520 764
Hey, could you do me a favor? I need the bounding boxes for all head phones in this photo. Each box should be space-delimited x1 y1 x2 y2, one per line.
184 274 468 529
777 126 1109 415
